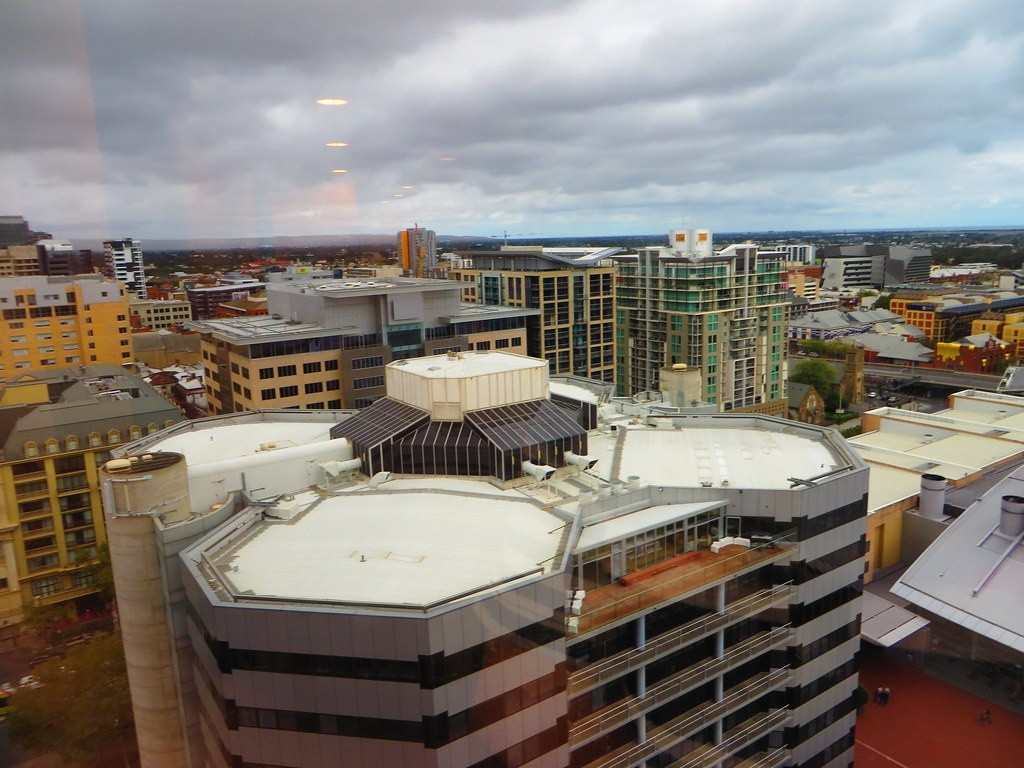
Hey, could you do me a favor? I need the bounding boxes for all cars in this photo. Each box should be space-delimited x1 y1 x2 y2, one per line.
796 349 820 358
30 651 65 669
881 394 890 401
868 391 879 398
888 396 900 403
